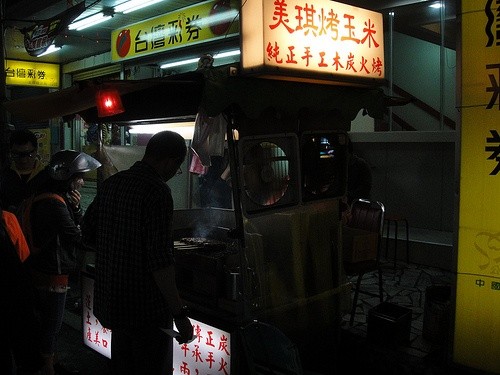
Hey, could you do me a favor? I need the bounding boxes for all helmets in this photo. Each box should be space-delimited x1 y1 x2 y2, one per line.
47 150 103 185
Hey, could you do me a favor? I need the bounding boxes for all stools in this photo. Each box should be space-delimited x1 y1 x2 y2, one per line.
381 214 410 273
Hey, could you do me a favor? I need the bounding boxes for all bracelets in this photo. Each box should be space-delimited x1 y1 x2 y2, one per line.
172 303 188 319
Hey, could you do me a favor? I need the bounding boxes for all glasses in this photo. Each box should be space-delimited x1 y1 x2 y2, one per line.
10 150 36 161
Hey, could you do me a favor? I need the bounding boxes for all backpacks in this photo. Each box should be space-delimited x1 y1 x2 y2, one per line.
1 193 67 271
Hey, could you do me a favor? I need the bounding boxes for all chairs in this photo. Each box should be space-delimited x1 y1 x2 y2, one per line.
332 198 385 325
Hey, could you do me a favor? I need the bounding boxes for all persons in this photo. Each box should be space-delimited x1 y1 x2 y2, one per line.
301 135 319 201
325 133 369 280
193 144 285 211
79 132 193 375
0 130 95 375
